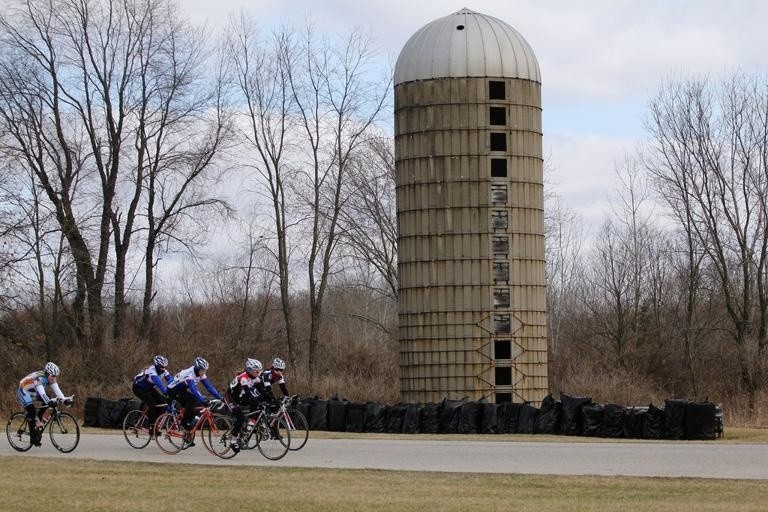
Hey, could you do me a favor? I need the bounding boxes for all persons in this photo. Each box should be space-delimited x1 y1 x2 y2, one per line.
132 354 174 436
224 357 272 452
17 362 67 446
249 356 293 441
166 356 223 430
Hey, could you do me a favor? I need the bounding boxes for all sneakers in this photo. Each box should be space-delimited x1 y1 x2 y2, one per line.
229 438 240 453
30 439 41 445
270 431 283 440
181 421 192 431
149 427 161 435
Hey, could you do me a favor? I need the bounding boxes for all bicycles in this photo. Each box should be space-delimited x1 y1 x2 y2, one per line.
6 398 80 453
122 392 309 460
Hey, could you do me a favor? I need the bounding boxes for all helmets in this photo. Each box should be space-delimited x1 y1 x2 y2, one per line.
271 357 286 370
45 362 59 377
153 355 168 368
193 356 209 370
246 358 262 372
